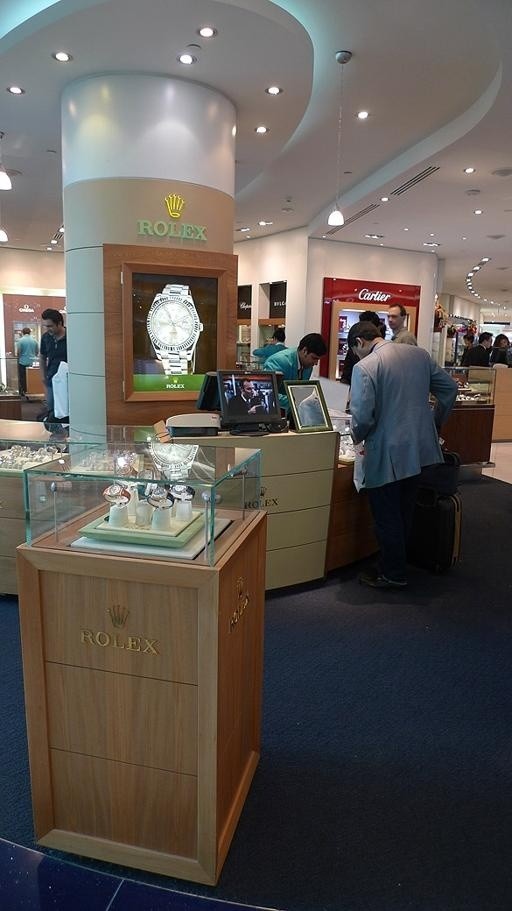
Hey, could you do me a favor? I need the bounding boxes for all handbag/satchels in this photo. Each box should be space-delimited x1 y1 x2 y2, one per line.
51 360 68 419
353 440 365 492
421 440 458 497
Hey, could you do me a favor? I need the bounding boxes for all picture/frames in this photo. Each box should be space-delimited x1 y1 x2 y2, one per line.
283 379 333 431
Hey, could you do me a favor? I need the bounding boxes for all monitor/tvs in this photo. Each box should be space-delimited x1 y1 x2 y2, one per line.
216 368 282 436
196 370 221 411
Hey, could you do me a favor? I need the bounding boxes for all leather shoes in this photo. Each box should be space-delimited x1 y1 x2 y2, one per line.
359 574 410 593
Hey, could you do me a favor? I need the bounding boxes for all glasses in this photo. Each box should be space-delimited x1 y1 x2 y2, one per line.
53 339 58 350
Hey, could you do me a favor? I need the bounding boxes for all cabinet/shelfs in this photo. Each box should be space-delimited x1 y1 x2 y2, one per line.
26 356 47 401
430 366 511 481
16 439 263 886
176 429 337 593
237 318 285 370
328 301 416 381
323 417 365 575
1 420 70 596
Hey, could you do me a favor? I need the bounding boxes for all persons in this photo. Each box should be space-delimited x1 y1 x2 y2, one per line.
461 334 475 366
37 308 67 424
15 327 39 396
348 320 459 590
228 378 265 416
340 310 381 383
263 332 327 421
253 329 288 366
385 302 418 347
461 331 493 367
490 334 512 367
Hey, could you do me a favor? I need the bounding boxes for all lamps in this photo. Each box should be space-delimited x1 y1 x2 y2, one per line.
0 230 10 242
1 132 13 191
328 50 352 227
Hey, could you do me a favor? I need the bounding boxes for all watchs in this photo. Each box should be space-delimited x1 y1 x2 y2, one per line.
147 442 199 484
101 484 131 504
147 486 175 509
145 283 205 377
169 484 195 500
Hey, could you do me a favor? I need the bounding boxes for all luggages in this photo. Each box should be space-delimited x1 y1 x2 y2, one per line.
416 489 462 573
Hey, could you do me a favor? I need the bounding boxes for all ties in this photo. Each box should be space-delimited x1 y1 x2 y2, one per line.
244 399 250 409
